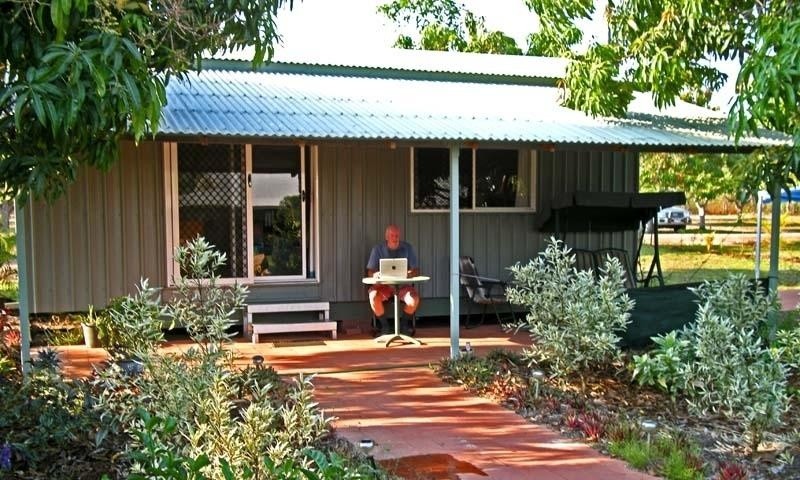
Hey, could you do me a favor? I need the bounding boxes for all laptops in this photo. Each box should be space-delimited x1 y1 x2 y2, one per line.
379 258 408 280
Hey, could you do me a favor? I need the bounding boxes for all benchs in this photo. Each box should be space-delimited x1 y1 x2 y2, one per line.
243 301 338 342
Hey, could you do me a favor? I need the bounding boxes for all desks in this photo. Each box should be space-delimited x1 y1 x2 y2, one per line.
362 275 431 348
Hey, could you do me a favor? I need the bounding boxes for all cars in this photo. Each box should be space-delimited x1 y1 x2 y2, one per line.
645 204 687 233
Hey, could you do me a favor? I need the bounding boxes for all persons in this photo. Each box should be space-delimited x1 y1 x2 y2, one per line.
366 224 420 335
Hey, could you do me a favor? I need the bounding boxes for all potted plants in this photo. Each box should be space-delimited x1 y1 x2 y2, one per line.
67 304 103 348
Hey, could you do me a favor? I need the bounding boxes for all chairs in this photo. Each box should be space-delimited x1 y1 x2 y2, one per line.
457 256 516 332
